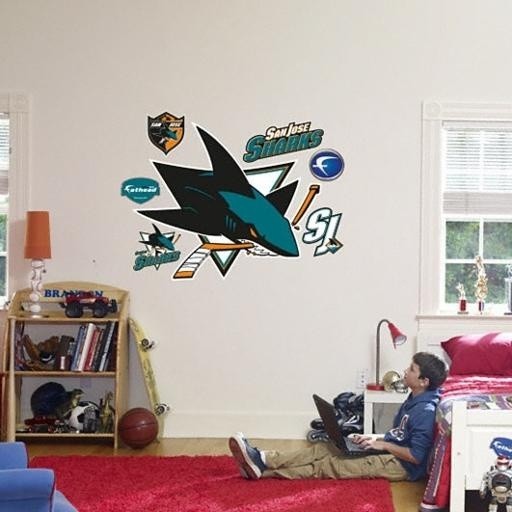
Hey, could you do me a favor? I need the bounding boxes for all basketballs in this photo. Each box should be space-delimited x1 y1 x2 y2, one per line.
118 407 159 449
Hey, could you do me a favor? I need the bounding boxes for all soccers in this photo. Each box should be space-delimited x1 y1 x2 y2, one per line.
66 401 99 433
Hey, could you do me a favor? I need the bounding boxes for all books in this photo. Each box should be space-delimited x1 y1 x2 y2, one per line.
70 320 117 372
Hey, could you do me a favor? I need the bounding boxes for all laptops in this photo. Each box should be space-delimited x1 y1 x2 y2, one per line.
312 393 383 457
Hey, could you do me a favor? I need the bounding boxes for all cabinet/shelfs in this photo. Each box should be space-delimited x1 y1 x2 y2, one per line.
7 281 130 450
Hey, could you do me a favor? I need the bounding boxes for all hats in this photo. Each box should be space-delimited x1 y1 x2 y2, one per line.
31 382 82 415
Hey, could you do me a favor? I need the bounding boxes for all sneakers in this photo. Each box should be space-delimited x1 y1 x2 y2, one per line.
228 431 268 480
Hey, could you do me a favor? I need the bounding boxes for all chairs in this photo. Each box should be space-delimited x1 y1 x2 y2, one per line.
0 441 79 512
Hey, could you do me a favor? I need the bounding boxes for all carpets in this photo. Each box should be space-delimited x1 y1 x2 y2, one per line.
28 454 395 512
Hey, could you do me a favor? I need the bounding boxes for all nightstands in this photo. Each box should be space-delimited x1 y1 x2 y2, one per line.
363 387 413 437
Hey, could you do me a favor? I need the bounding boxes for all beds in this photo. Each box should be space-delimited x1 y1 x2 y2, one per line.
415 314 512 512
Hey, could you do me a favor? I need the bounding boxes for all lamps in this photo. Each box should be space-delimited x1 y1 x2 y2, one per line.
23 211 52 313
367 318 407 390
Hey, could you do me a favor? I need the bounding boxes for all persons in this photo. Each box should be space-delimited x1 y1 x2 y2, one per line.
229 353 446 481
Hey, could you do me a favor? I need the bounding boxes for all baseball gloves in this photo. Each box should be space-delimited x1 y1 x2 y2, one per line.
22 335 60 370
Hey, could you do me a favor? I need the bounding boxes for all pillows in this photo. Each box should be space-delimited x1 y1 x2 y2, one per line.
441 332 512 377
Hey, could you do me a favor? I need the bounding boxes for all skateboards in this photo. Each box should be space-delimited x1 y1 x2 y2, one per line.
128 318 170 444
